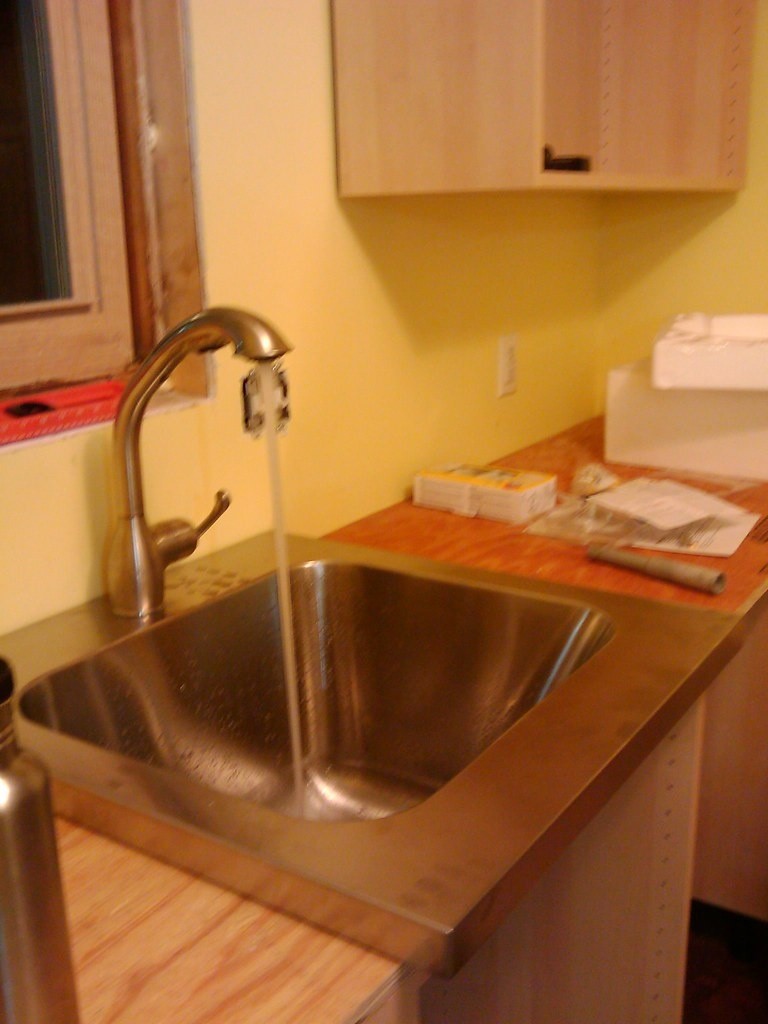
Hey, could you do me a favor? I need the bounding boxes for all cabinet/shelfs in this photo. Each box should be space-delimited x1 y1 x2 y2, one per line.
333 0 753 198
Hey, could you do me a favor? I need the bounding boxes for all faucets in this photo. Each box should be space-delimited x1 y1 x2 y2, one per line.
106 308 292 620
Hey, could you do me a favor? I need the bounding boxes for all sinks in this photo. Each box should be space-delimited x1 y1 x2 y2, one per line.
14 543 622 887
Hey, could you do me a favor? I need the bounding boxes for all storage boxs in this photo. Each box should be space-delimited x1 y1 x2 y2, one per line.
603 362 768 482
652 314 768 392
